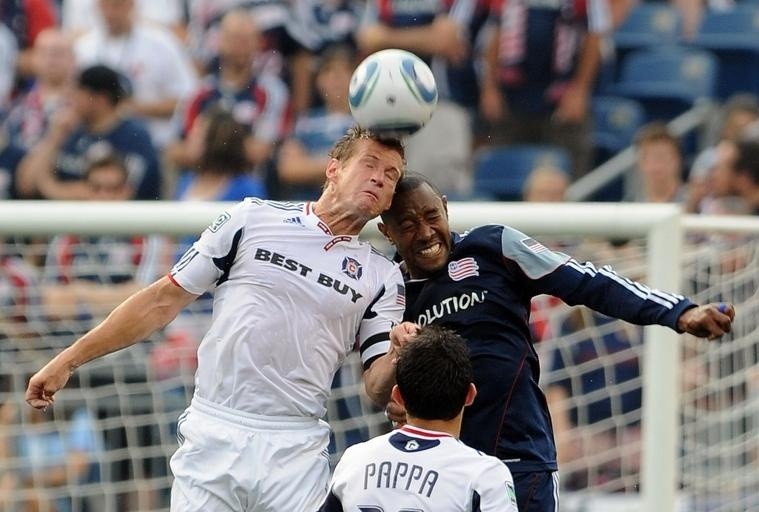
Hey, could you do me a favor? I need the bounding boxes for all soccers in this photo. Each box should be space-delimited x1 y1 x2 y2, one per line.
349 49 438 134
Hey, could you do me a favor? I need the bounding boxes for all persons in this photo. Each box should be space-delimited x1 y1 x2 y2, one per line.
1 1 759 511
